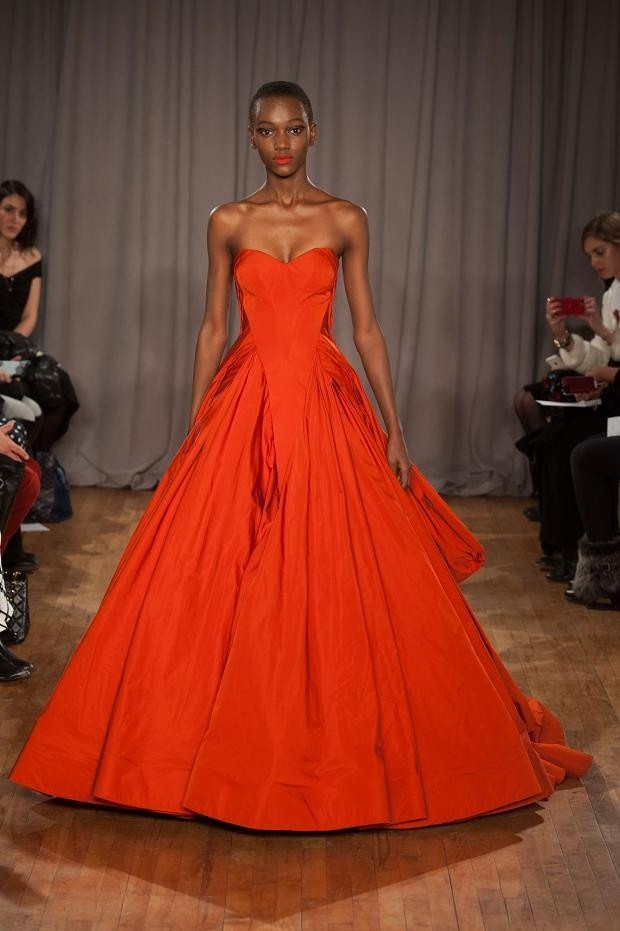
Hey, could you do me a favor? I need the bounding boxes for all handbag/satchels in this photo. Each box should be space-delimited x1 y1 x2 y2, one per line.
22 450 72 521
1 572 29 643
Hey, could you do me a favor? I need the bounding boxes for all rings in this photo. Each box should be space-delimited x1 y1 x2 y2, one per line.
551 309 558 317
546 296 551 303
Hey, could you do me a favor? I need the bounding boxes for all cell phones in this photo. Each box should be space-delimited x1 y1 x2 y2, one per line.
561 376 598 393
553 298 584 315
545 354 564 370
0 360 31 377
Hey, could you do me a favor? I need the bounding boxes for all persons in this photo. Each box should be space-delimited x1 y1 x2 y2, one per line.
11 79 595 835
513 209 620 611
0 179 46 682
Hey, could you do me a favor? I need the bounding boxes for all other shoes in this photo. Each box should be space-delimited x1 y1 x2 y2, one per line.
524 503 573 581
0 643 34 681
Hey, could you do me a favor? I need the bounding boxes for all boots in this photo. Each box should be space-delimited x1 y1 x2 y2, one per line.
563 534 620 607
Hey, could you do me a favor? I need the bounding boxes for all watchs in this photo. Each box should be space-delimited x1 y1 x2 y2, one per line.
553 330 570 348
